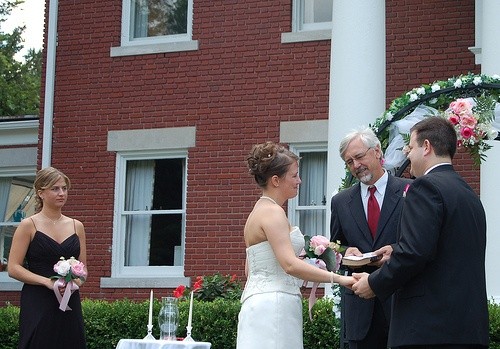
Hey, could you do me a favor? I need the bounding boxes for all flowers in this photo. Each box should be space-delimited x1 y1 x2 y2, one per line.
373 71 500 169
301 234 344 321
51 255 88 312
403 183 410 197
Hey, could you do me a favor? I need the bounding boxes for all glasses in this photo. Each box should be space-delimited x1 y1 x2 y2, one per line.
346 147 372 168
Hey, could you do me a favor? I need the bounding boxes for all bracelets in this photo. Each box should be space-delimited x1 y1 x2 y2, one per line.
331 271 334 286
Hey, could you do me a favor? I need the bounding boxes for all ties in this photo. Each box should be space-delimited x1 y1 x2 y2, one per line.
366 187 381 241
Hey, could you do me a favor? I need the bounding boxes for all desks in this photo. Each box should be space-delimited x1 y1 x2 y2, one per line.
116 339 211 349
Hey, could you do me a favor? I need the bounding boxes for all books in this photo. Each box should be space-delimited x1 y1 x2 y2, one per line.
342 252 383 266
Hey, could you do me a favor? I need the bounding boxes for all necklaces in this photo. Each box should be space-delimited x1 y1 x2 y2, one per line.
40 212 63 225
260 196 276 204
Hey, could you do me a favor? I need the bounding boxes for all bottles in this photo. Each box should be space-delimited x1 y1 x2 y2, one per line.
158 296 180 341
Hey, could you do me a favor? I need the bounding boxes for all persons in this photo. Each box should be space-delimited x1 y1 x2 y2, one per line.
330 127 416 349
8 166 88 349
352 116 490 349
237 141 358 349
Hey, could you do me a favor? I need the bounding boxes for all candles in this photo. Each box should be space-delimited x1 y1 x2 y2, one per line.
188 291 193 326
149 290 154 324
